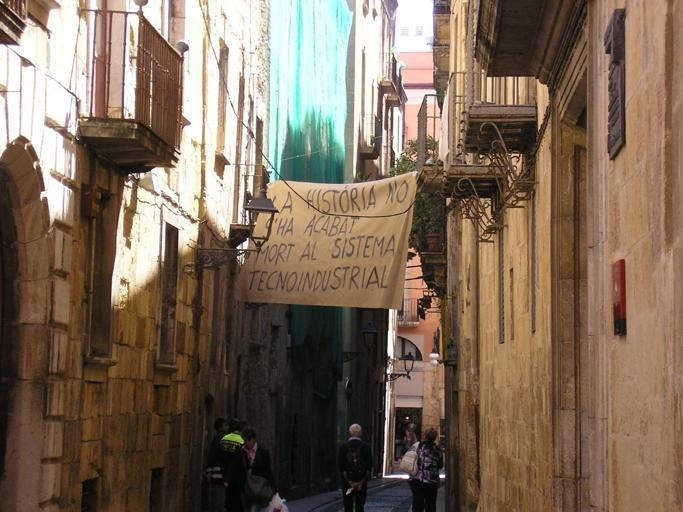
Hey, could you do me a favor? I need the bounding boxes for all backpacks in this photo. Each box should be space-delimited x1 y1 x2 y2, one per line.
400 442 420 476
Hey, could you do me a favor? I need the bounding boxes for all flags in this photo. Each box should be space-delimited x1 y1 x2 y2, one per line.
238 167 420 309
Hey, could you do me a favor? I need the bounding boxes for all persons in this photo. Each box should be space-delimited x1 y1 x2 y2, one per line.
400 428 443 512
403 423 416 449
207 417 230 473
220 418 246 512
336 423 372 512
239 430 276 512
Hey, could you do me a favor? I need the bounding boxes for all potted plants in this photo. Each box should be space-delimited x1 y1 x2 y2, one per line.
413 190 446 252
